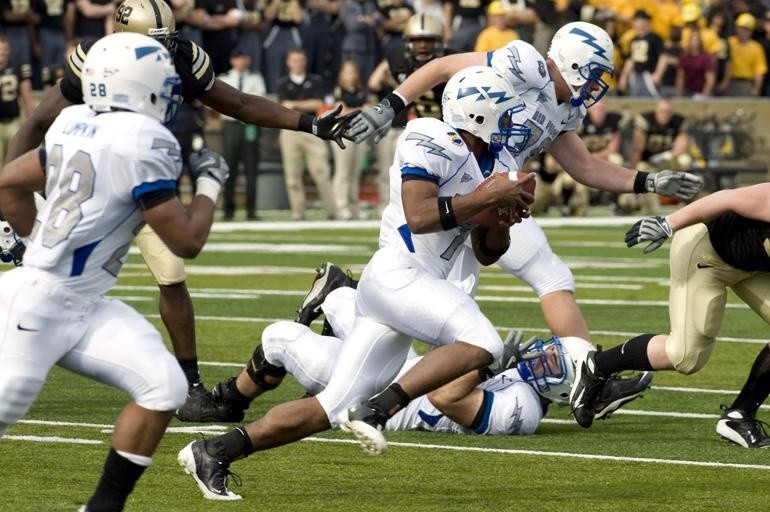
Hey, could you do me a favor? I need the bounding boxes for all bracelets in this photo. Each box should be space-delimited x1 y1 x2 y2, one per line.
19 237 28 246
438 195 455 234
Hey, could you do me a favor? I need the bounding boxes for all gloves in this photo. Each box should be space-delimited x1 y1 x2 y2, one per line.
190 148 229 202
485 326 542 373
634 168 705 199
624 215 674 256
299 92 409 148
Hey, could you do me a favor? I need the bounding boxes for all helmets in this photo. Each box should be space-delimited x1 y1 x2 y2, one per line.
517 335 599 404
441 65 531 151
550 22 617 110
82 30 183 133
112 0 179 59
399 14 446 57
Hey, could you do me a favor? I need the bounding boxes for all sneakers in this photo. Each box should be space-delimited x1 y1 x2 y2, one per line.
296 259 344 326
716 402 770 450
593 371 653 419
569 350 597 428
176 382 249 423
288 200 376 220
0 220 23 264
339 400 387 458
176 434 247 502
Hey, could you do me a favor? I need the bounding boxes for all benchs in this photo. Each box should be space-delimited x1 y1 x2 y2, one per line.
675 158 767 190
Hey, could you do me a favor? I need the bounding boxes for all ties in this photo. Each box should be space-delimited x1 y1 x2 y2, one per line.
239 75 243 92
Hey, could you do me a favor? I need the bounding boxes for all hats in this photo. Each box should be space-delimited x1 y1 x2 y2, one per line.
488 2 505 17
682 4 759 55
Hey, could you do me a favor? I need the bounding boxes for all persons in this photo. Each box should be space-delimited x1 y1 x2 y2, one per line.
176 67 546 498
177 286 600 436
0 0 359 402
570 183 770 449
2 35 231 511
299 23 703 419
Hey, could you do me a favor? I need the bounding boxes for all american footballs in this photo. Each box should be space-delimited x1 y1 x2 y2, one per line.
466 171 534 225
